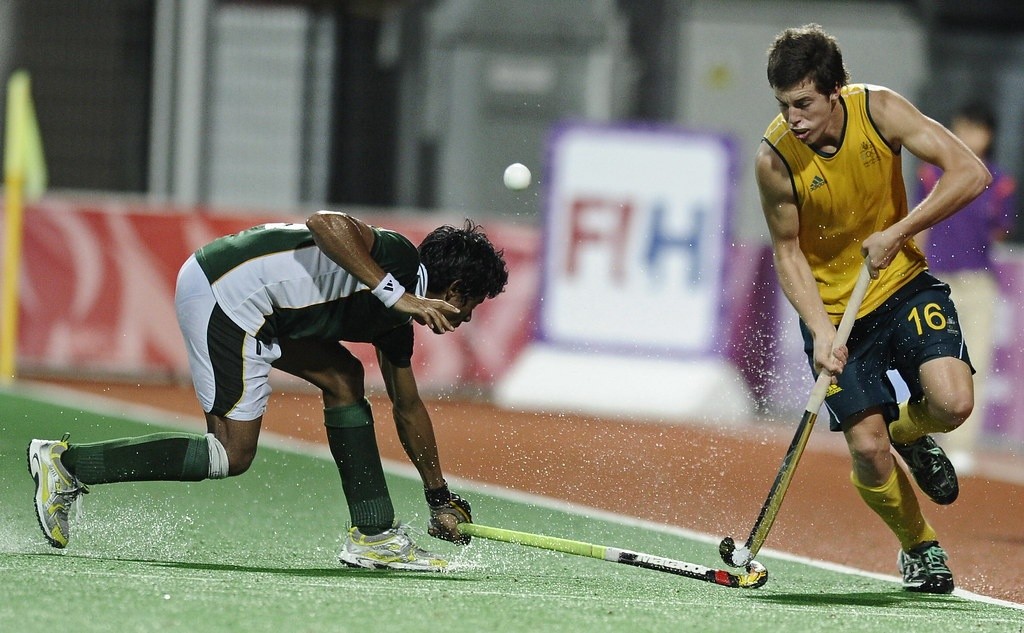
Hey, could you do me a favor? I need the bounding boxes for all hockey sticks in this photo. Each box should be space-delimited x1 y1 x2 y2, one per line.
719 251 871 568
439 521 769 592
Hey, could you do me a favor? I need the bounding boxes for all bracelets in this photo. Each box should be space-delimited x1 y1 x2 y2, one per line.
424 478 452 506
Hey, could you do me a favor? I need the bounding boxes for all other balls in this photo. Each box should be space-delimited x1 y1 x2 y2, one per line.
503 164 531 191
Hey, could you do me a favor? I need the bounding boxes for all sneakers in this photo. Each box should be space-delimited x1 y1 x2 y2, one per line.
339 518 449 574
27 432 92 548
897 541 954 595
888 402 959 505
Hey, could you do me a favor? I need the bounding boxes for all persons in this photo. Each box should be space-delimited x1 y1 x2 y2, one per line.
755 21 1014 593
27 211 508 575
